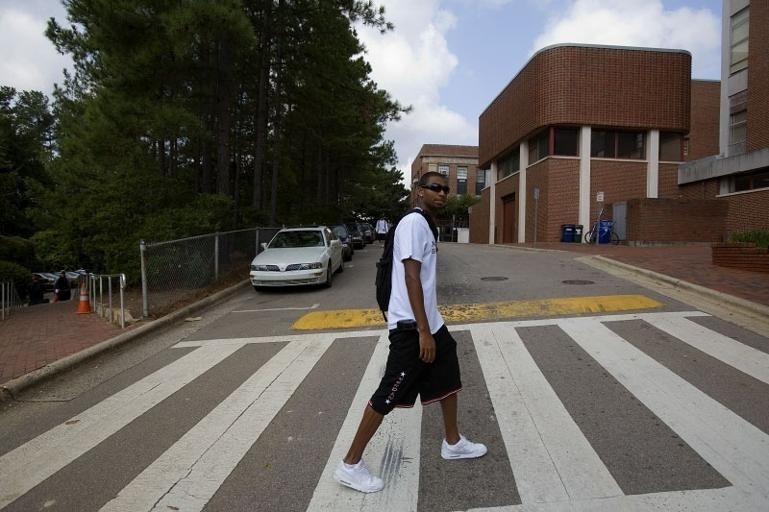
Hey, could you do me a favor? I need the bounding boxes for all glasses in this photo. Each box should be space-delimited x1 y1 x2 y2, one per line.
421 183 450 194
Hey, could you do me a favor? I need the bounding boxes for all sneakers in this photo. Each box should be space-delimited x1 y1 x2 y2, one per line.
333 458 384 494
440 432 487 460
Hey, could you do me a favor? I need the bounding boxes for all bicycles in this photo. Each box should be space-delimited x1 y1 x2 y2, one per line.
585 221 619 245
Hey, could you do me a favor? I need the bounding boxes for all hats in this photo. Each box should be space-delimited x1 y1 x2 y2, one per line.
58 270 65 274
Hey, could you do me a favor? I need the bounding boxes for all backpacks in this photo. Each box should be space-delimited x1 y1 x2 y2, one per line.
375 208 438 310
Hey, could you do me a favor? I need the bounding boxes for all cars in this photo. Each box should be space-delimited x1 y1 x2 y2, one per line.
249 225 345 291
327 224 378 260
31 269 95 289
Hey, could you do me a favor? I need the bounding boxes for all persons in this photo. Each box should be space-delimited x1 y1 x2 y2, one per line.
23 271 79 307
331 172 487 496
372 216 393 247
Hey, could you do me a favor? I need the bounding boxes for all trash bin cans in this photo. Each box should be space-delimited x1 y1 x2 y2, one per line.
595 220 612 244
560 224 583 243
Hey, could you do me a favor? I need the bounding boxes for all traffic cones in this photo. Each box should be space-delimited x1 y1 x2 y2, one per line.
75 282 93 314
